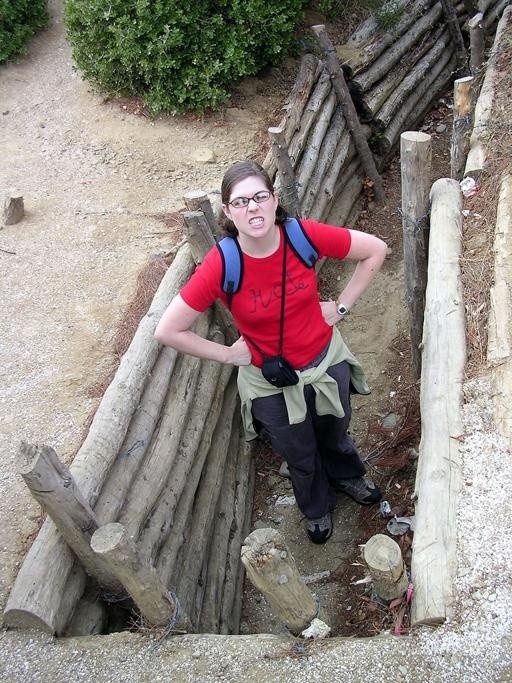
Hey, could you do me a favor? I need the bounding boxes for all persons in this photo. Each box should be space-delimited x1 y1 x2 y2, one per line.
154 161 388 545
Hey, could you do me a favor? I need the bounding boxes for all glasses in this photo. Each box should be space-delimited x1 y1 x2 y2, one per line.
228 190 273 208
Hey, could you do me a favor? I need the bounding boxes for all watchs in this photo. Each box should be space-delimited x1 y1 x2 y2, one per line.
335 299 348 315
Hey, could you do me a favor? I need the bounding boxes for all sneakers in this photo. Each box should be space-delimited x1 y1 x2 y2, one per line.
307 510 332 545
335 476 385 506
